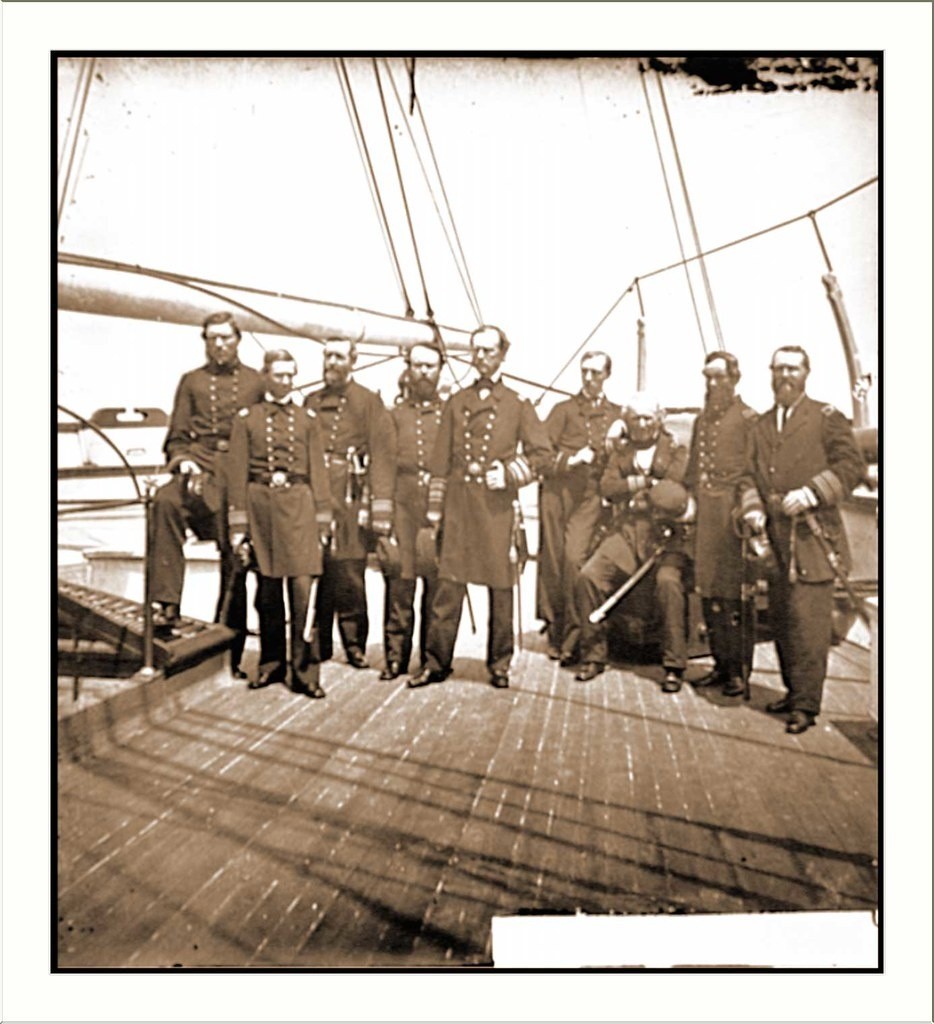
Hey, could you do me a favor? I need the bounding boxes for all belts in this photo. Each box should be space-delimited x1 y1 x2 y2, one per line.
248 472 311 489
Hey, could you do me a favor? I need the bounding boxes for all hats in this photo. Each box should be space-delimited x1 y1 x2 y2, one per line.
648 479 689 519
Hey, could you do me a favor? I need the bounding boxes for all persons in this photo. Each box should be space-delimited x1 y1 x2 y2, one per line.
379 342 453 681
574 394 696 694
301 335 398 669
535 351 640 665
226 350 333 700
144 313 267 679
734 345 868 734
407 326 554 690
681 352 758 696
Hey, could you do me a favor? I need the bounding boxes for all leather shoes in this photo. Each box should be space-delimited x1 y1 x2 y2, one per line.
378 662 407 683
575 662 607 679
408 666 448 688
344 652 370 670
763 697 797 714
723 679 747 696
247 667 285 689
290 681 327 699
490 671 511 689
787 717 816 735
661 666 684 694
692 669 722 688
